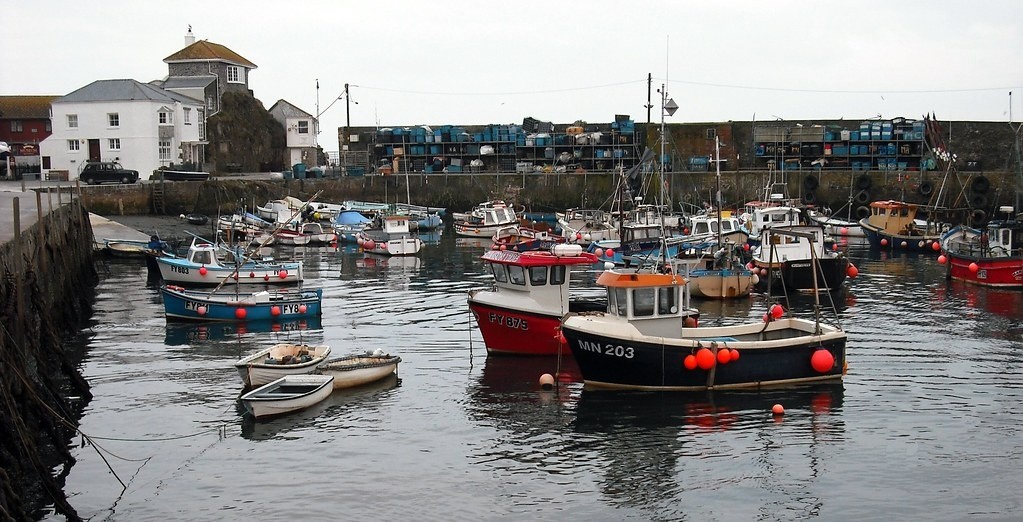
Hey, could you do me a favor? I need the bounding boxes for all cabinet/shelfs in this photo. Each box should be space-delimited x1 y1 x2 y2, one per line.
753 122 926 171
374 127 644 175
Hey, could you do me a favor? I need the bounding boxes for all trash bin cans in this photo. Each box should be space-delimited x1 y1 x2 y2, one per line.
283 170 292 180
310 167 322 177
293 162 306 178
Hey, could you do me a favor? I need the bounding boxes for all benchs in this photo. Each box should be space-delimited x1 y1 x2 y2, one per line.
45 170 69 182
255 394 304 396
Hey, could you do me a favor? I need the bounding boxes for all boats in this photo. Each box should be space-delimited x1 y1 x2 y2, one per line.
185 212 209 226
233 334 331 389
240 374 335 421
807 169 866 238
157 169 211 183
152 83 857 356
106 240 158 258
555 156 847 395
858 197 974 252
937 220 1023 291
314 346 402 390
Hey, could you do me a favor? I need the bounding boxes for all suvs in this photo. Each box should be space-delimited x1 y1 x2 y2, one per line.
79 162 142 185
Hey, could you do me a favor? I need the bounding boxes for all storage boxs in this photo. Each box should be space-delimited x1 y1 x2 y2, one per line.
271 151 370 180
374 114 633 174
754 117 924 172
689 156 709 171
